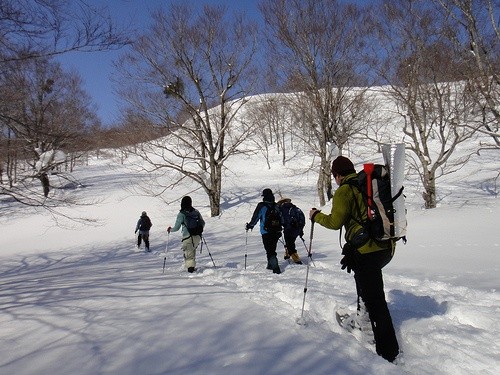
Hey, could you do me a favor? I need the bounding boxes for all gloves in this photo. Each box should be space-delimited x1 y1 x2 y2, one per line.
135 231 137 234
167 226 171 234
245 224 250 230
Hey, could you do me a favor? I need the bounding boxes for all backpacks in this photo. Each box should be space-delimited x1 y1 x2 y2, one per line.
261 202 282 232
339 163 407 255
283 203 306 230
180 209 203 236
140 215 151 231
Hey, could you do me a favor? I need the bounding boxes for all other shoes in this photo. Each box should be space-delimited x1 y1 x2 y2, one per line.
188 267 194 273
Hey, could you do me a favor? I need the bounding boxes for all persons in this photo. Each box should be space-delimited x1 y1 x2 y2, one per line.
246 188 303 274
167 196 201 273
135 211 152 252
309 156 403 365
43 174 49 197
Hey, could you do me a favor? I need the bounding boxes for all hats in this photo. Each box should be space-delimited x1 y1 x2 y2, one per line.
142 211 146 215
261 189 274 196
277 197 291 203
331 155 356 177
